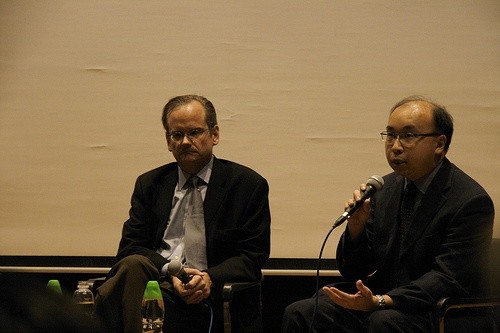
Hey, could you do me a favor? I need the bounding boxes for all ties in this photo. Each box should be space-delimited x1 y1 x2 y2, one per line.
183 176 208 272
393 183 417 288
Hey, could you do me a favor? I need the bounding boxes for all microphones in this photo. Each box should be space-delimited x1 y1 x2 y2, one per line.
332 175 384 227
168 261 210 308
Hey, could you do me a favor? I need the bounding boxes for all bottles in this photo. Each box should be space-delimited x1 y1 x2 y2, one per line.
72 280 95 321
47 279 63 297
141 280 164 333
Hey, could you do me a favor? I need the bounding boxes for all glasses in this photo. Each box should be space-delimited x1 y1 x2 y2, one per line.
167 129 207 142
379 130 437 142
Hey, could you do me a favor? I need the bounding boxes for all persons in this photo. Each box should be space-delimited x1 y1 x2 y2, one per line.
278 95 495 333
97 93 272 333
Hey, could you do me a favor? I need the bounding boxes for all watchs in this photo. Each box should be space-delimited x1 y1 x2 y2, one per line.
376 293 387 310
